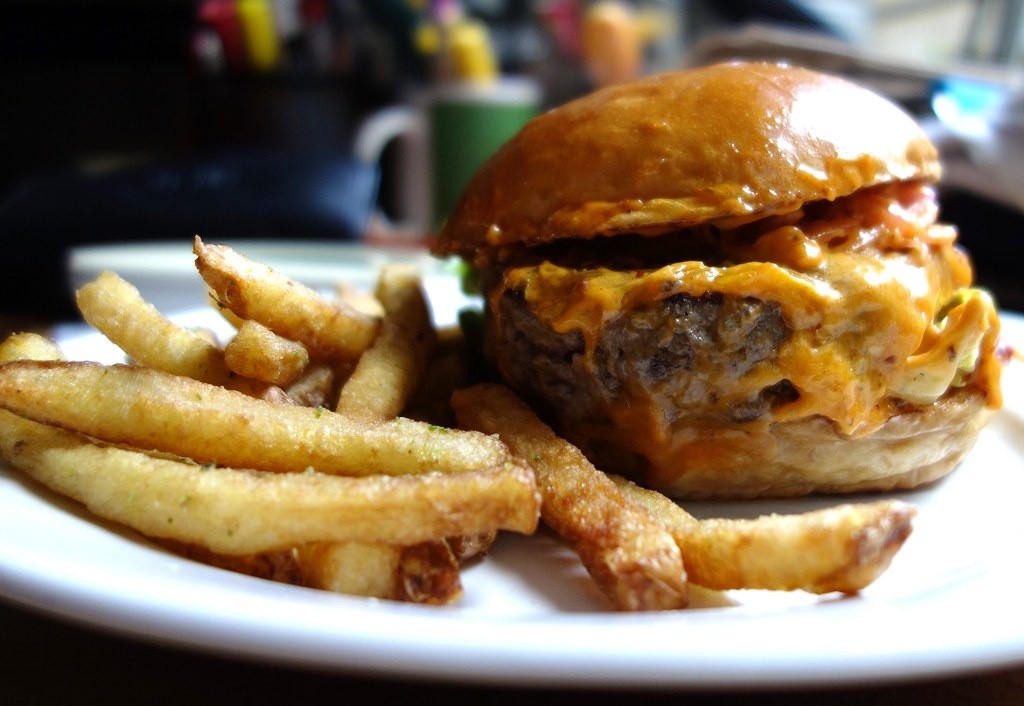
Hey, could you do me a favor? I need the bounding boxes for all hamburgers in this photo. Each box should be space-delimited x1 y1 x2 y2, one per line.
429 63 1001 499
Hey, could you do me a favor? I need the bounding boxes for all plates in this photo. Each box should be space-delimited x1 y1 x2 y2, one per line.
0 310 1024 688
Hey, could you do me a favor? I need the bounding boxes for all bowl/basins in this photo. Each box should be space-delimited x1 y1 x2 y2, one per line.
66 237 456 314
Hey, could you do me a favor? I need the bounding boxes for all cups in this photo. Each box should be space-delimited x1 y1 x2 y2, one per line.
349 87 430 245
428 82 544 224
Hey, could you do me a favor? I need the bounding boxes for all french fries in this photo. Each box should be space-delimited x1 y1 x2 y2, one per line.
2 235 918 611
